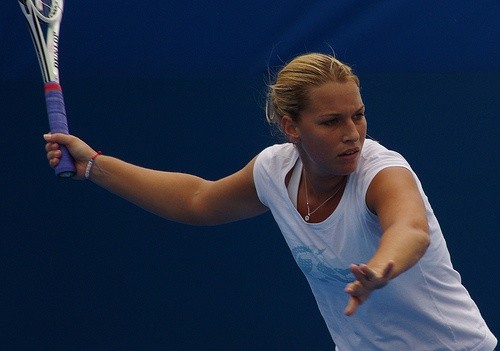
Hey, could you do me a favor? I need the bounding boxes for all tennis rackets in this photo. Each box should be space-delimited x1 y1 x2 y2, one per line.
14 0 77 180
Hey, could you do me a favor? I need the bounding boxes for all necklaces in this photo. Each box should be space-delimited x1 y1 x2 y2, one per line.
302 167 345 221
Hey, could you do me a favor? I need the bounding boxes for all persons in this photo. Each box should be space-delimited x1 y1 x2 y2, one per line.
44 53 500 351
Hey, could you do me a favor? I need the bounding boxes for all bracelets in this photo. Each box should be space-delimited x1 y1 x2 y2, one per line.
84 150 103 179
